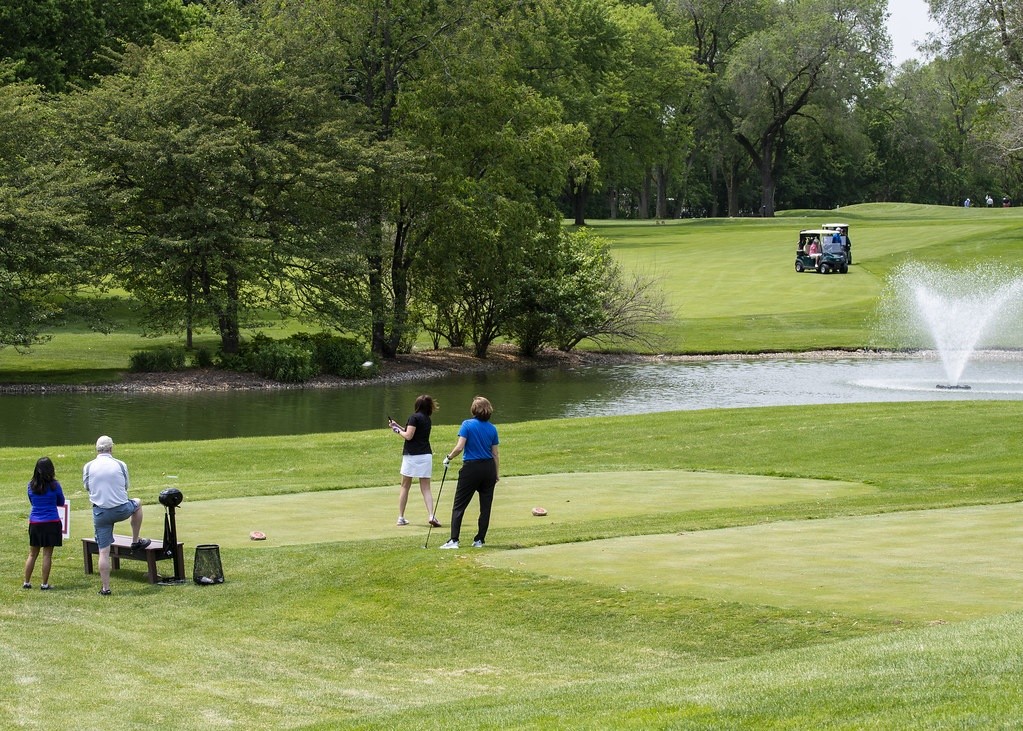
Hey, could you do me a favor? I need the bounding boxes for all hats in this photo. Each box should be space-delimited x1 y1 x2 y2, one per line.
96 436 114 450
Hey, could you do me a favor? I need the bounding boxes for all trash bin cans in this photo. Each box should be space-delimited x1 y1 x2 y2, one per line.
193 544 225 584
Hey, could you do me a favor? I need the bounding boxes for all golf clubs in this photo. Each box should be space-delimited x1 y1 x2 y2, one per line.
361 361 392 423
421 462 450 549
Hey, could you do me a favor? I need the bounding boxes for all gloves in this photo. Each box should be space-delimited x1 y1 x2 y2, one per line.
443 457 452 468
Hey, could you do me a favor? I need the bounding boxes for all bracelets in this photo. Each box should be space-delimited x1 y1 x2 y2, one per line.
447 455 452 460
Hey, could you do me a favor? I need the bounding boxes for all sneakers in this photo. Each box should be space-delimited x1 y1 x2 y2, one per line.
429 517 441 527
397 518 409 525
99 586 111 595
131 538 151 553
439 539 460 549
41 584 53 590
815 264 818 267
23 584 33 589
472 540 482 547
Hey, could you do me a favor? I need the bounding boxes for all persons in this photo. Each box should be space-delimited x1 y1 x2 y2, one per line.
964 198 970 207
804 228 851 268
388 394 441 527
1002 195 1010 207
439 395 500 550
83 435 152 595
23 457 65 590
987 196 993 208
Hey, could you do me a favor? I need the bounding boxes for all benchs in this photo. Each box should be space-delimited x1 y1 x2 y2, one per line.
82 534 185 584
803 245 823 258
